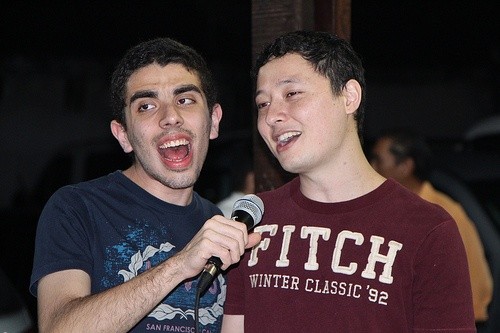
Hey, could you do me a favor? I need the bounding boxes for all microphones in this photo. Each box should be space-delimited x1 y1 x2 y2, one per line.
198 194 264 293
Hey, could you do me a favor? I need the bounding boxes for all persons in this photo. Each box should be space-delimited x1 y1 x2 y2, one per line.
29 38 262 333
220 31 478 333
217 148 256 219
368 130 493 322
